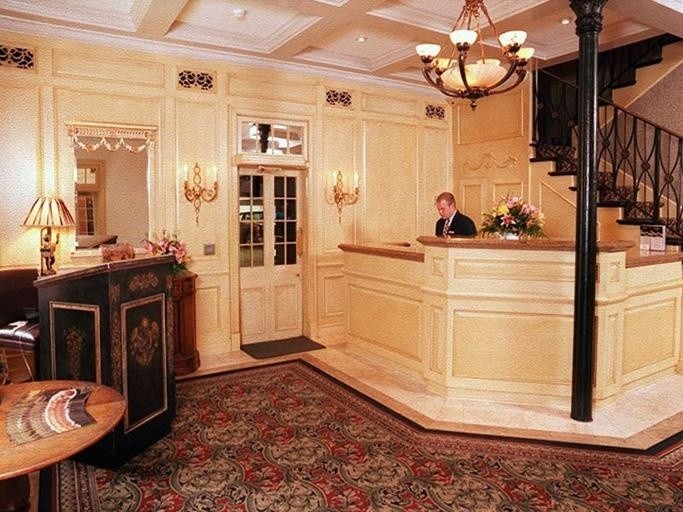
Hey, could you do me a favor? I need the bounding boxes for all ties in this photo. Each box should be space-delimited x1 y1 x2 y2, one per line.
443 219 451 236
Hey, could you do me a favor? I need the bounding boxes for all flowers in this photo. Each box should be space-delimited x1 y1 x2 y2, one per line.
473 196 550 239
139 234 191 273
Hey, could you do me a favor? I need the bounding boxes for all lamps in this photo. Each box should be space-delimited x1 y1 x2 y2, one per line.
183 163 219 227
19 195 76 277
415 0 539 112
330 170 368 225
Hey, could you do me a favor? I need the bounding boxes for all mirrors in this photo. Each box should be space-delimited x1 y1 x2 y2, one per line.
64 120 161 261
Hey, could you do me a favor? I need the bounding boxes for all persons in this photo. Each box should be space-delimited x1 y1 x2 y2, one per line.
435 192 477 238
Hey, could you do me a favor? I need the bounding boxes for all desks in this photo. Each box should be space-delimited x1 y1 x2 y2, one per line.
0 378 127 512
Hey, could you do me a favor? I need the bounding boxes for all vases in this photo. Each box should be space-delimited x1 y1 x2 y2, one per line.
503 227 522 245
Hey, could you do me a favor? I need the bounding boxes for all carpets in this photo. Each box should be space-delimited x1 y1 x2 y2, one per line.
34 358 683 512
239 334 328 359
